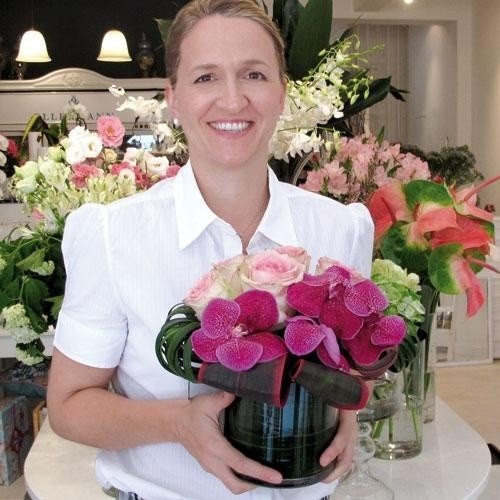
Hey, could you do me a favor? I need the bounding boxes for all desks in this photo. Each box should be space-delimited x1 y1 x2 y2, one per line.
21 390 492 500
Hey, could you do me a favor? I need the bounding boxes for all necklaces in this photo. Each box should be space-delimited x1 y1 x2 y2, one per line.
236 193 268 239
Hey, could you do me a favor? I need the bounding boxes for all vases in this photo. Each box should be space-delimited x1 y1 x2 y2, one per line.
361 365 438 461
221 395 343 486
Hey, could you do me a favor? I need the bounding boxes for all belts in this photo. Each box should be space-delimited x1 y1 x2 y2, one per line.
101 484 142 500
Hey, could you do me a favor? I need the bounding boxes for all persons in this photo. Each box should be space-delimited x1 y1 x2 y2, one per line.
46 0 376 500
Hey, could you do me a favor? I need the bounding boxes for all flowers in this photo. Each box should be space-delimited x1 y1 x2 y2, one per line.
0 0 499 398
